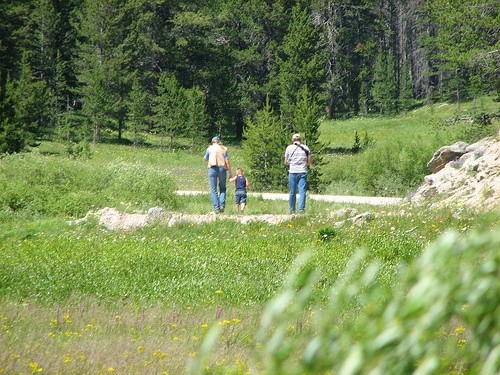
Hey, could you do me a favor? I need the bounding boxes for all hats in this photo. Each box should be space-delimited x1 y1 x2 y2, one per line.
293 134 300 138
212 137 220 141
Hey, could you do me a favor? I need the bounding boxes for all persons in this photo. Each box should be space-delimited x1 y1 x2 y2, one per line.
285 134 311 214
229 168 251 212
203 136 233 214
